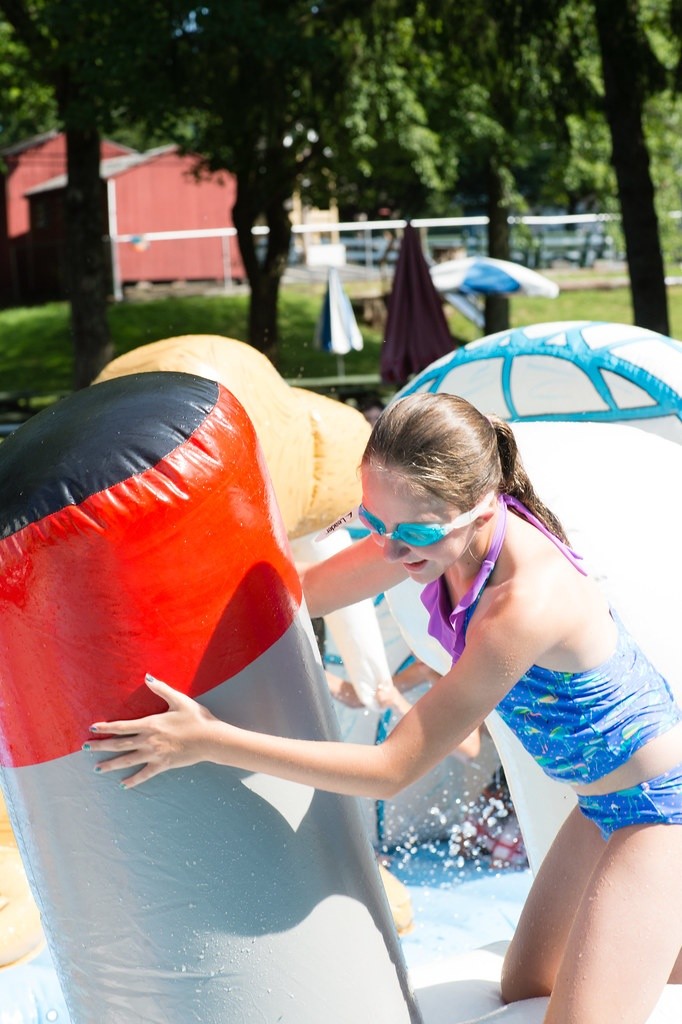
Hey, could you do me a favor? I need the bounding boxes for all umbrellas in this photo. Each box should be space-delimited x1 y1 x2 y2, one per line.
432 255 560 299
378 218 457 390
312 266 364 375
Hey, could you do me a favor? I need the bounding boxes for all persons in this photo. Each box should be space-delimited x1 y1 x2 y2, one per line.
357 398 385 426
82 393 682 1024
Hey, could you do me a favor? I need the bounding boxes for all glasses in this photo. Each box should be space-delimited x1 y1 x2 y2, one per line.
357 505 450 548
